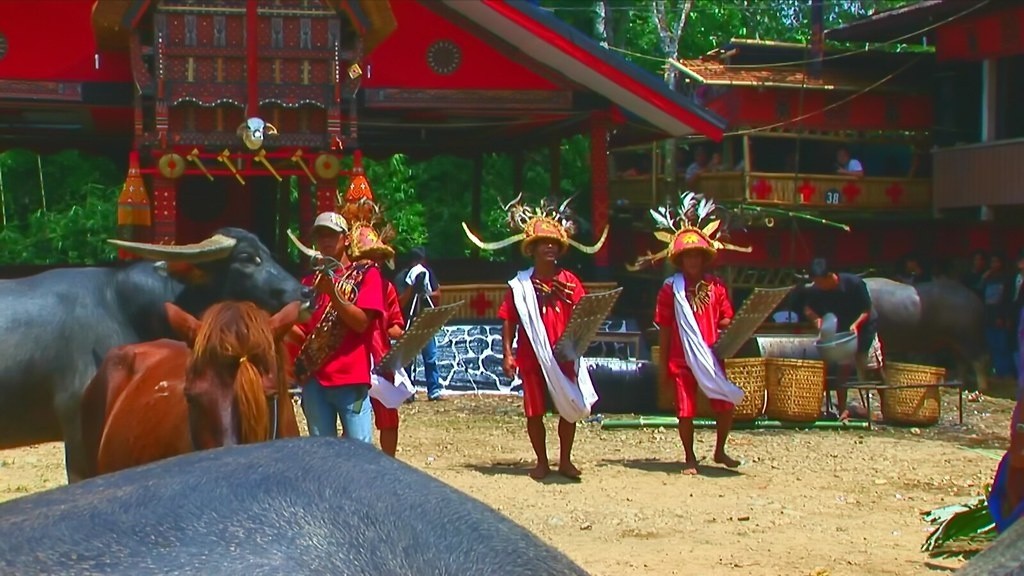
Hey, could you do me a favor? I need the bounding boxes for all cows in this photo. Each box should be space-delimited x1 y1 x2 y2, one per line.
797 270 989 394
0 229 314 490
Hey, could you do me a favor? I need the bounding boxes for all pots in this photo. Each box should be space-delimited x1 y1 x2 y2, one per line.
813 332 858 362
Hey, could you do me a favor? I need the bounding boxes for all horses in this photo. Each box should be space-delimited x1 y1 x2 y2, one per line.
83 297 300 477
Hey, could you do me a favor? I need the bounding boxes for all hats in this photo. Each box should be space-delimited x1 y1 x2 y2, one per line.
286 222 395 263
312 212 348 235
463 193 610 258
627 192 754 271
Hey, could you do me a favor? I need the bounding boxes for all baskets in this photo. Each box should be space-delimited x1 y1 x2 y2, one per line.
767 358 826 421
884 360 945 426
721 358 764 418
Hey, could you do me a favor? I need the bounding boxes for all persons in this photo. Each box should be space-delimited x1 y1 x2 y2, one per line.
616 139 865 185
656 227 742 475
803 258 873 420
498 215 586 477
904 249 1024 377
285 212 449 457
